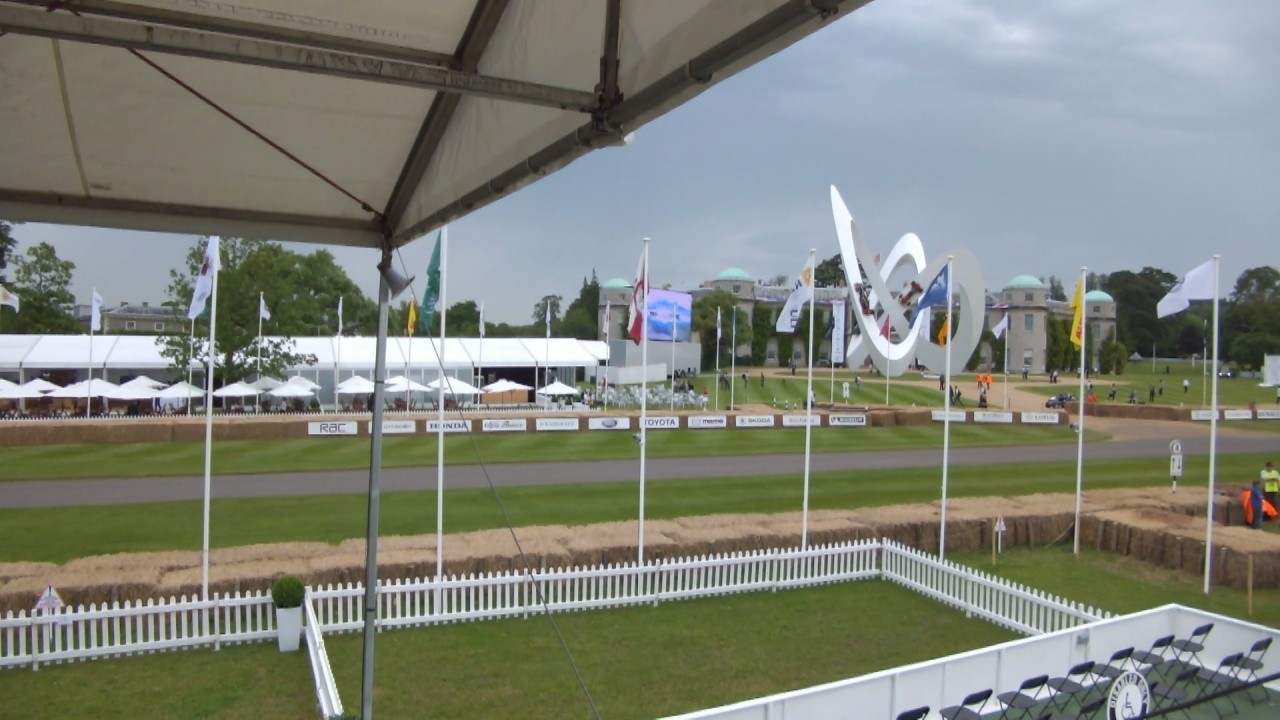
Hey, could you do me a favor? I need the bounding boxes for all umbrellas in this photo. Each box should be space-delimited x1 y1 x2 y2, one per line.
0 375 577 409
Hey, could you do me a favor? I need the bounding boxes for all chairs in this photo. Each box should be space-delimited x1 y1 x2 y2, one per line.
0 381 709 422
896 621 1274 720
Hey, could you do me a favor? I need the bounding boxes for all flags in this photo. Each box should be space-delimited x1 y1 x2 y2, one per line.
909 265 947 329
546 305 551 339
338 300 342 334
1156 260 1215 318
92 293 102 331
0 284 18 313
776 256 812 332
420 232 441 329
673 308 677 341
992 316 1007 340
603 303 610 333
717 308 736 340
408 303 416 334
479 308 486 338
188 235 222 320
879 318 888 338
627 249 646 344
1069 275 1085 351
260 297 270 320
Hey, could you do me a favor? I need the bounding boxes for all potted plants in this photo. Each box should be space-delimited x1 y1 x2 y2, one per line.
270 574 306 652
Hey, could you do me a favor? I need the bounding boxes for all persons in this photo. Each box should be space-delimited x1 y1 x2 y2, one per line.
1251 461 1280 528
790 355 1189 408
689 370 764 410
